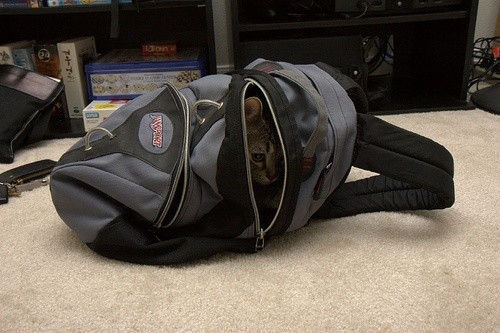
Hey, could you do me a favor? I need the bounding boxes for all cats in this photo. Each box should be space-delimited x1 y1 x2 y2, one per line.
244 96 286 214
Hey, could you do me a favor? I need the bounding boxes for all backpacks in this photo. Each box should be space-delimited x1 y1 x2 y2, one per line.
50 57 454 264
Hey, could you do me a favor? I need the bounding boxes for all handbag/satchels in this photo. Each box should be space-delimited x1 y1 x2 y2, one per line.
0 63 64 164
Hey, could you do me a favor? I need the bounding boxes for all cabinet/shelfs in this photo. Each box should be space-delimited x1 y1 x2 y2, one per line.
1 0 479 140
